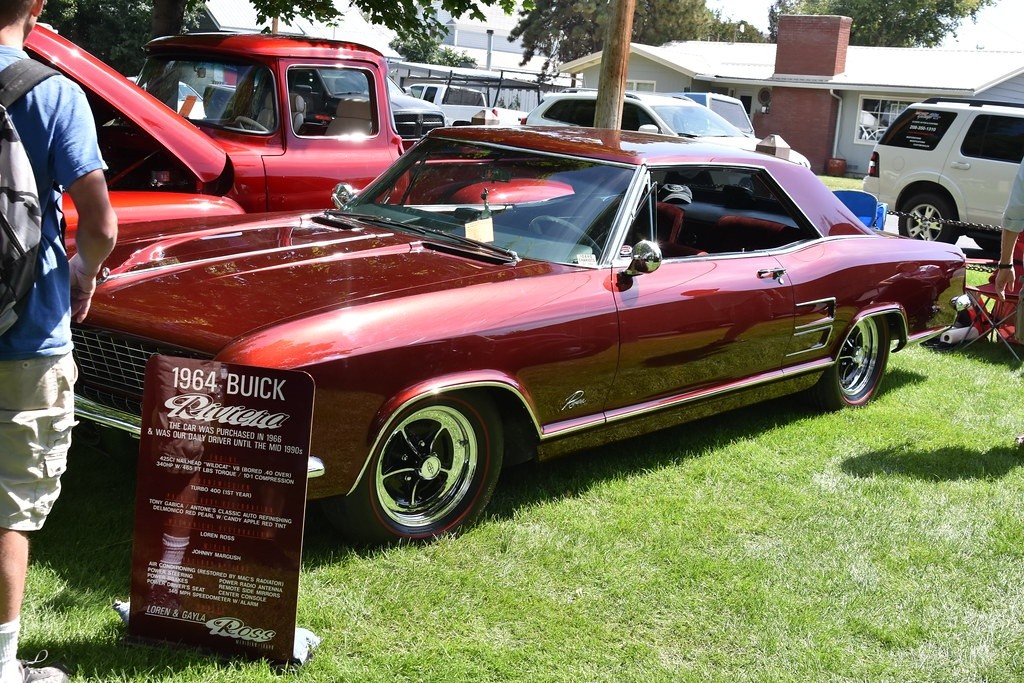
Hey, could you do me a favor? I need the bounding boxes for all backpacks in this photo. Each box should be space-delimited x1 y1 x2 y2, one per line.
0 58 62 339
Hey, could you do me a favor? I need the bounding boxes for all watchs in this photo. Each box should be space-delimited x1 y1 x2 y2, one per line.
998 261 1013 268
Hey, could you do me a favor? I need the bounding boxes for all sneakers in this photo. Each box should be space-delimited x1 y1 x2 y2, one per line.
139 580 181 634
16 649 67 683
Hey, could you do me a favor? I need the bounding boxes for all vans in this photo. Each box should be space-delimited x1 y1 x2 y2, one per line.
409 81 541 129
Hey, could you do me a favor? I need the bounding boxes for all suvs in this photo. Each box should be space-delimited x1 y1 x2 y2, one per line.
862 97 1024 251
519 87 811 191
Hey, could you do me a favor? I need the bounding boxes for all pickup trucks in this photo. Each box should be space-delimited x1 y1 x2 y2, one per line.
203 73 445 139
21 24 553 283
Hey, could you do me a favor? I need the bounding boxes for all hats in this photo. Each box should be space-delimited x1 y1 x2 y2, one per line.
657 183 692 204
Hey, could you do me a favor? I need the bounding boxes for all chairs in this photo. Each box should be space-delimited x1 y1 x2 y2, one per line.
950 232 1024 361
631 204 794 260
323 95 373 136
257 91 304 135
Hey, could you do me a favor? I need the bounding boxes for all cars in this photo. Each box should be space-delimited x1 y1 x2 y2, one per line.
69 125 972 545
102 76 206 126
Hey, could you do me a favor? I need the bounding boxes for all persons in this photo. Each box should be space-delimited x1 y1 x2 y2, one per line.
0 0 118 683
994 157 1024 445
145 354 224 608
